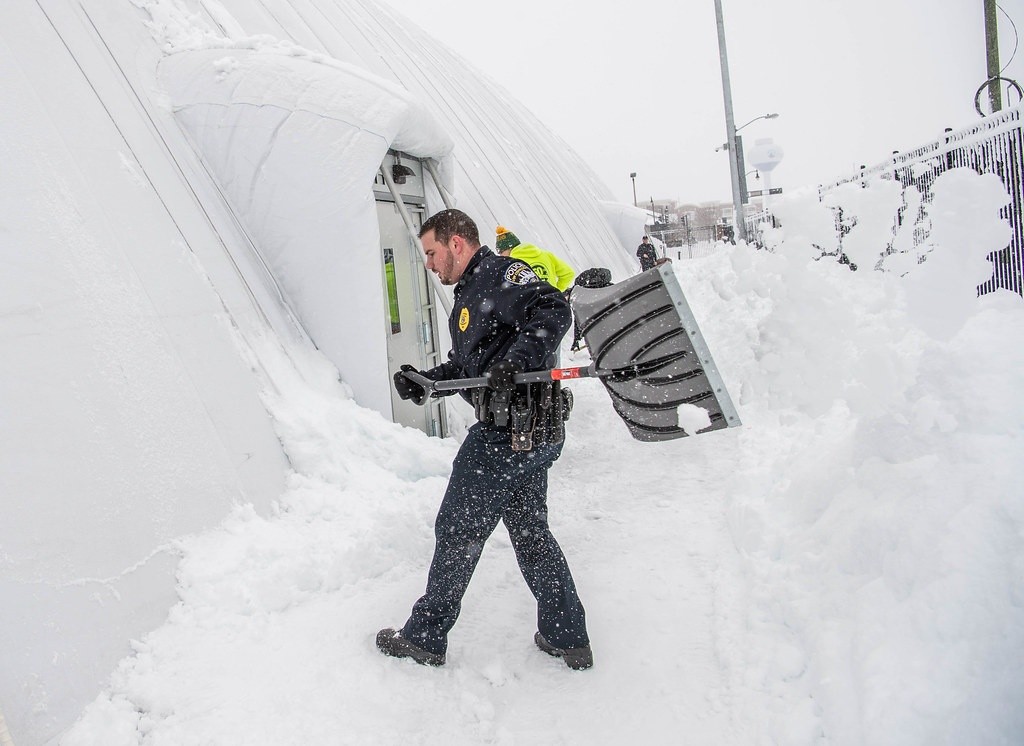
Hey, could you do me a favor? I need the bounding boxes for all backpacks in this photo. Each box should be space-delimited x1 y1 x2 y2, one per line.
575 268 611 288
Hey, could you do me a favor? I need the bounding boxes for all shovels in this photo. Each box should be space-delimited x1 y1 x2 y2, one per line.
399 261 744 444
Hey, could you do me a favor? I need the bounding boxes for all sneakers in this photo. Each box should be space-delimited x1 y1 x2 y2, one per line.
534 631 593 670
376 629 446 667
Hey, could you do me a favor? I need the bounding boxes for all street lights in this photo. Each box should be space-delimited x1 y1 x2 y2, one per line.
715 112 780 237
630 173 636 207
649 197 655 224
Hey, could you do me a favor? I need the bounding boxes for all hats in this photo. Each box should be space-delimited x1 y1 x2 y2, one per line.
496 225 521 254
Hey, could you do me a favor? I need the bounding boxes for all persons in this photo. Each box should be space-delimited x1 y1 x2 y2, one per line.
496 225 575 293
374 208 594 673
636 235 657 272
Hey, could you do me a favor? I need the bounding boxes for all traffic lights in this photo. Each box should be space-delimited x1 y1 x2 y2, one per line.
681 216 686 226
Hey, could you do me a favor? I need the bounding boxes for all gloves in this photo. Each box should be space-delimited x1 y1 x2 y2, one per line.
571 341 580 351
394 365 429 400
487 361 521 393
